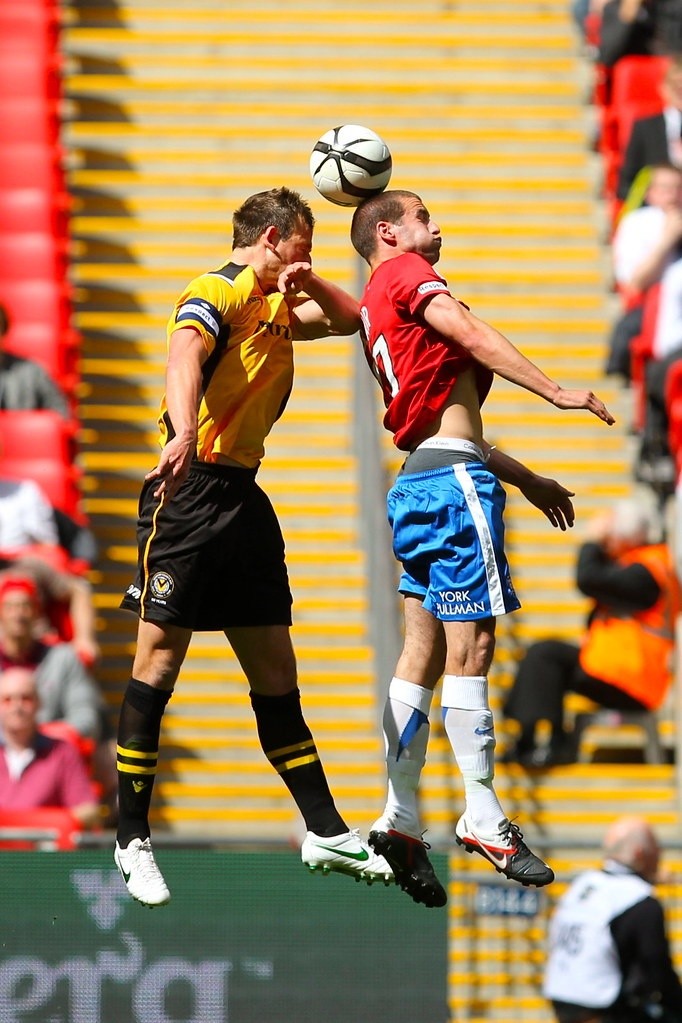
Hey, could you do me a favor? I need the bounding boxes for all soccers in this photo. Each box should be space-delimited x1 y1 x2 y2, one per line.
309 126 394 210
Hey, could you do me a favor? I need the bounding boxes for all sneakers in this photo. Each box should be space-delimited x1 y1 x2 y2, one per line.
366 814 447 908
301 827 396 887
453 812 554 887
114 837 171 909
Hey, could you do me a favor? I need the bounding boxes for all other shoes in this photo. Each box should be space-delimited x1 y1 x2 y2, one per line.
497 738 536 763
527 743 575 765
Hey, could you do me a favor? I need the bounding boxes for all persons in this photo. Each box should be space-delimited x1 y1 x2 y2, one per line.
0 578 107 739
546 822 681 1023
0 480 60 550
0 307 69 419
495 491 681 766
0 667 103 825
115 189 394 905
575 0 681 503
350 190 617 910
0 556 102 659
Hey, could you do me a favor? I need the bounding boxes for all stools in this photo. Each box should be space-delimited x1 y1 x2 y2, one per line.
568 702 664 767
